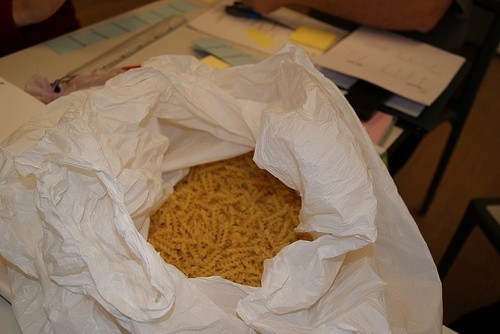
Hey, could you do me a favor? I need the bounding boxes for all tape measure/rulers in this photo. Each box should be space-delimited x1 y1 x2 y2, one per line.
65 13 188 84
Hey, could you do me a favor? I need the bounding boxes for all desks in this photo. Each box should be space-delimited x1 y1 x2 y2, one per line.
0 1 500 219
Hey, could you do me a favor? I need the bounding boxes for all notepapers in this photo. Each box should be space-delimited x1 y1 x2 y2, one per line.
289 24 339 52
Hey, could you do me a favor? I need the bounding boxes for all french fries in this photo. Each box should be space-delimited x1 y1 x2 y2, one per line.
147 151 314 288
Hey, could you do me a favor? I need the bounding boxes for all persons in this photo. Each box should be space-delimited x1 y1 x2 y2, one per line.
0 1 80 60
242 1 453 34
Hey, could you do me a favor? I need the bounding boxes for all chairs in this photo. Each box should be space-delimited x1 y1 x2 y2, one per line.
435 197 500 279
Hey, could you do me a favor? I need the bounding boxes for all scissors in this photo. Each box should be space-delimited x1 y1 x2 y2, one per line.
224 1 294 31
